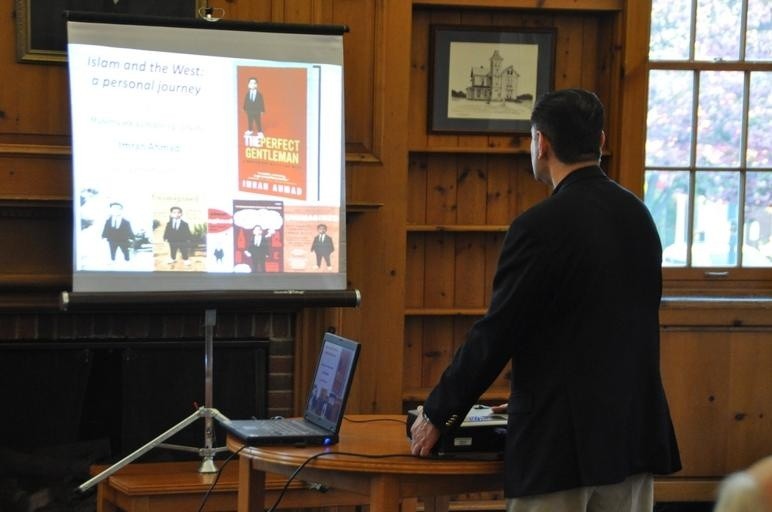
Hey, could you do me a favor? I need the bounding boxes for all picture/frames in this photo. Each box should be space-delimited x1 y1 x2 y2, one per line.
427 21 560 137
13 0 207 67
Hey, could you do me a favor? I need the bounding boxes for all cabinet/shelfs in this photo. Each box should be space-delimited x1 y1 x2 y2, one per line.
398 145 615 404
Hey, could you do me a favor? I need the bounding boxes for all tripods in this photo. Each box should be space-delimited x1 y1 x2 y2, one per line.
70 308 328 496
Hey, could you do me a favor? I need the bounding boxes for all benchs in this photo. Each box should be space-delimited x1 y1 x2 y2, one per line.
89 460 371 512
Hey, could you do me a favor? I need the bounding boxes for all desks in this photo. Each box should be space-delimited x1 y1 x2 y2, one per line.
227 414 506 512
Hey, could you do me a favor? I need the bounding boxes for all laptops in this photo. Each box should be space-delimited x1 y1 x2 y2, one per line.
219 333 361 445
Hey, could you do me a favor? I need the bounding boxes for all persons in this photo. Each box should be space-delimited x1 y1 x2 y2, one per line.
310 224 336 270
162 207 193 264
101 204 138 261
246 226 269 273
713 457 772 512
243 77 266 137
408 90 684 512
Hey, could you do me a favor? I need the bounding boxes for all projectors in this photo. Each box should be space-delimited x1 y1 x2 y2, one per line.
405 405 508 455
